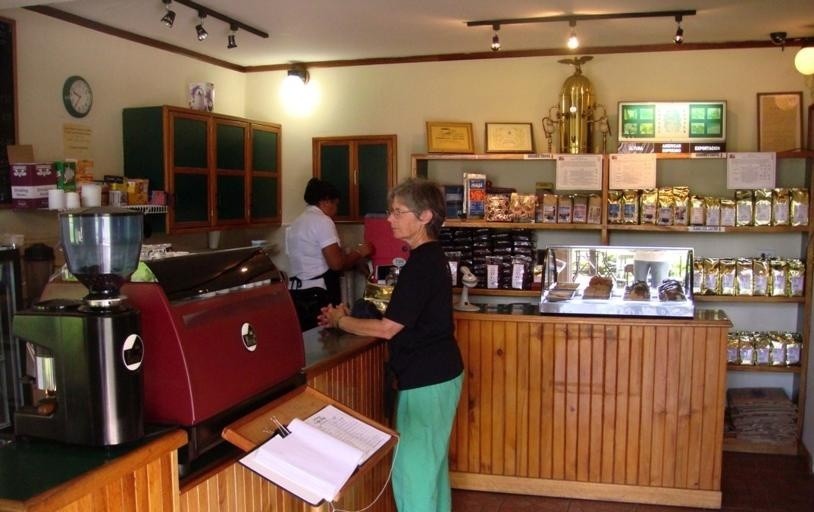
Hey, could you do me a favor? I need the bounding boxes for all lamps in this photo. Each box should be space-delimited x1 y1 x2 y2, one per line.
159 0 244 53
278 61 311 111
486 11 688 55
795 35 813 78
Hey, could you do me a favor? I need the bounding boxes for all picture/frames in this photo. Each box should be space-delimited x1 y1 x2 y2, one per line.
616 99 726 145
755 91 804 159
425 119 475 155
483 121 538 154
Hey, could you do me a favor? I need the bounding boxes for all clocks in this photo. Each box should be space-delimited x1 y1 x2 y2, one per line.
62 75 94 118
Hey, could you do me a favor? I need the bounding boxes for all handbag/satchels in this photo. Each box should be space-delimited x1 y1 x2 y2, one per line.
351 298 382 319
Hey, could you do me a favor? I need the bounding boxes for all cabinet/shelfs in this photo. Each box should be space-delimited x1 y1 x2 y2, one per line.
122 106 283 232
410 149 811 461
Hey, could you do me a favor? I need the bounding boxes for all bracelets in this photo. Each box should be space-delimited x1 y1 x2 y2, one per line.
335 315 344 329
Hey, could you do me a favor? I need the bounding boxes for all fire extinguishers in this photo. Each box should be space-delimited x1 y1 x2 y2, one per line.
365 264 378 284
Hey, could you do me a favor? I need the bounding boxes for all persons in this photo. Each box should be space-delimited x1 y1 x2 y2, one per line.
285 175 375 334
317 177 466 512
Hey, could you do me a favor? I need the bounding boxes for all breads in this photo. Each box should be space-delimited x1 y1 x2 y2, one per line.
548 276 685 301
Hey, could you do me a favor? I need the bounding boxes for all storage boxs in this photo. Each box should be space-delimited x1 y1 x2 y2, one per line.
7 142 62 209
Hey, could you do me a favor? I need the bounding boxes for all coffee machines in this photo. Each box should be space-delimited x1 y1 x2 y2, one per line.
0 210 149 447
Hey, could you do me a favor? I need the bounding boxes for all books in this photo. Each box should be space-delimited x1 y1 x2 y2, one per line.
462 172 487 219
236 404 392 506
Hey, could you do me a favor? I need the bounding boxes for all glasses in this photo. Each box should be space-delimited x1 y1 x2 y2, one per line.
386 208 411 216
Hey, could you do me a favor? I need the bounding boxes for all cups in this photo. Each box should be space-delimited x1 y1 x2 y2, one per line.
48 181 102 212
208 229 220 248
